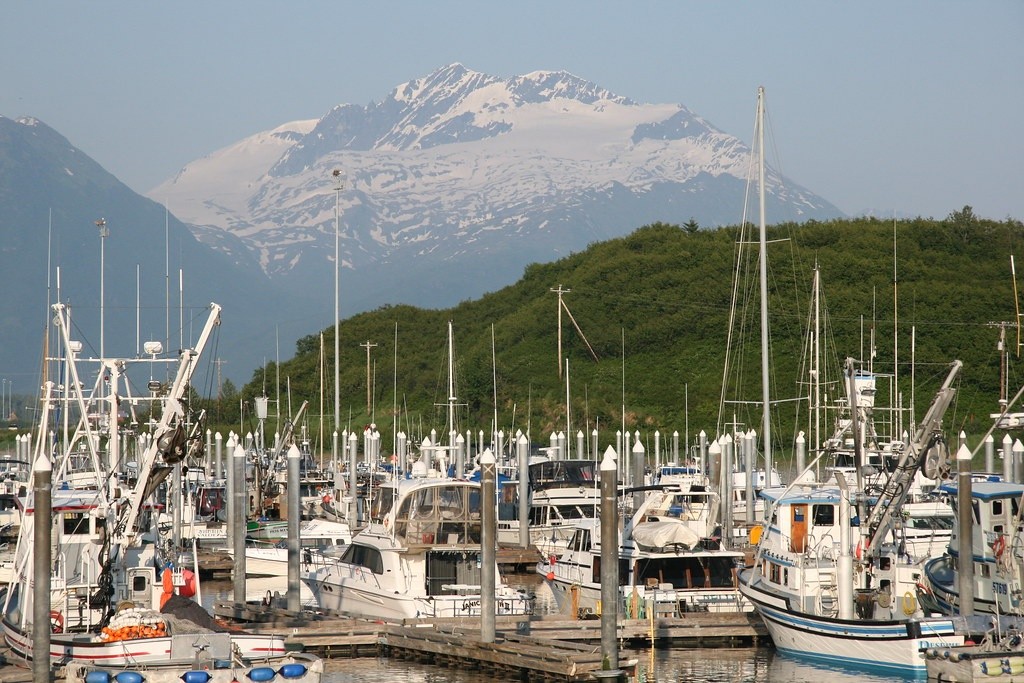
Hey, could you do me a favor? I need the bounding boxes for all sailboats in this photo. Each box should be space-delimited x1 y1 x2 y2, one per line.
1 84 1023 683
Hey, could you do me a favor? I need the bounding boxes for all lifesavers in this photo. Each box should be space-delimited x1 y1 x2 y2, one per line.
993 537 1004 559
50 611 65 634
